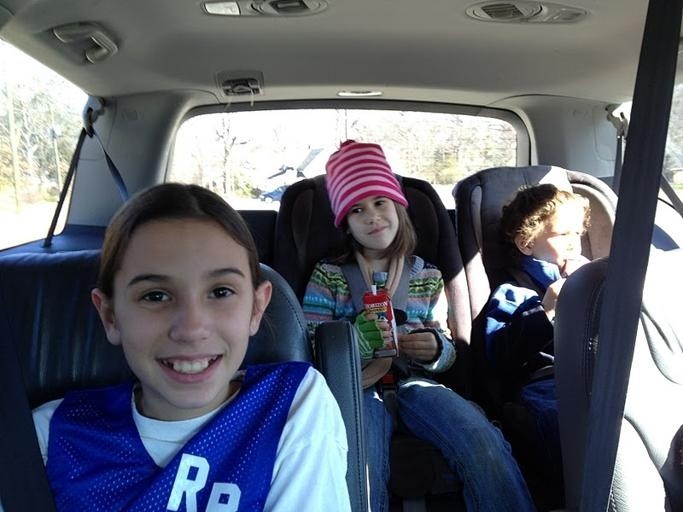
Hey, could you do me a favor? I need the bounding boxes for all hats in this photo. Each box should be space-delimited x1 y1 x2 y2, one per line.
326 138 409 227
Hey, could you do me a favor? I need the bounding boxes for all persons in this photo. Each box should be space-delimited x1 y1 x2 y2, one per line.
302 139 536 512
482 182 593 466
28 182 353 512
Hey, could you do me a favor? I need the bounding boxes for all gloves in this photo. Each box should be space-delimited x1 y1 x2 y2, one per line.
354 312 385 359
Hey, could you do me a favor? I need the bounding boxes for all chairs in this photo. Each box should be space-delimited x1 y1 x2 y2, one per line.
1 165 683 512
